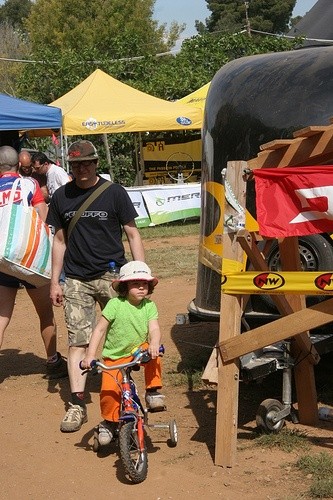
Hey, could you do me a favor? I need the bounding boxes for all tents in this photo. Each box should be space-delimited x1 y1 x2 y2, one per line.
0 69 213 183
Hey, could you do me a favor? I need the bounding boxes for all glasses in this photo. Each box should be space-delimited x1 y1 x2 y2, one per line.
2 162 19 169
23 163 33 169
70 160 94 167
34 164 45 170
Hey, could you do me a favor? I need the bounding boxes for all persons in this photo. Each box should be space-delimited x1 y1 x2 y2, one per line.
0 146 68 375
80 261 166 446
45 140 147 433
17 151 73 288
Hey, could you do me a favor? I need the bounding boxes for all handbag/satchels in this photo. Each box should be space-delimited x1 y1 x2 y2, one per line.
0 191 55 287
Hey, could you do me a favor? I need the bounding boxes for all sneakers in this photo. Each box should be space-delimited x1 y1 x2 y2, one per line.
45 353 68 378
61 402 88 431
144 392 166 411
98 422 115 445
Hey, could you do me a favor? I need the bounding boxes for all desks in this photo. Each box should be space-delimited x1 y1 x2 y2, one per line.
123 182 201 229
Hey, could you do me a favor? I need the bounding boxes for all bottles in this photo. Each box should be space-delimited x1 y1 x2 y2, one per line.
108 262 120 274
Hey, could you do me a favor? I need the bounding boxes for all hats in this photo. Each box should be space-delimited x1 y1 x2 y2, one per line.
112 260 159 297
68 140 99 162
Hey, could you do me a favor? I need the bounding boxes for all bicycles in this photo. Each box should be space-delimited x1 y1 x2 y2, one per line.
78 344 177 487
